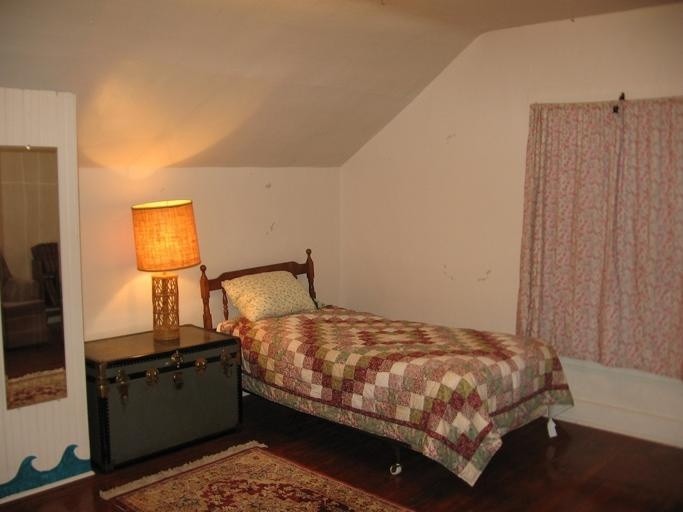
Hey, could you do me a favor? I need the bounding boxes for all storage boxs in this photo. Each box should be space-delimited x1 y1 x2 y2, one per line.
83 324 243 475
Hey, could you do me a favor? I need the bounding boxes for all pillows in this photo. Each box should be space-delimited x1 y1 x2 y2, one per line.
221 271 319 323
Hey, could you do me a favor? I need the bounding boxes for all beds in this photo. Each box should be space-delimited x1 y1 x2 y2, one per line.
200 248 575 488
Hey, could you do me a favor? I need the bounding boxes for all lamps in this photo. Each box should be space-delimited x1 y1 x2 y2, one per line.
131 198 201 341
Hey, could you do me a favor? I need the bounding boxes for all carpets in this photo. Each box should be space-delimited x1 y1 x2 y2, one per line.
6 367 67 410
100 440 415 512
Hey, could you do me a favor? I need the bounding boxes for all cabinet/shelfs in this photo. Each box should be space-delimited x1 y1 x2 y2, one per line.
0 86 94 504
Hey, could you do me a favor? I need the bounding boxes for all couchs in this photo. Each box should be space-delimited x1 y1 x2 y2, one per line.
31 242 61 306
0 256 47 350
